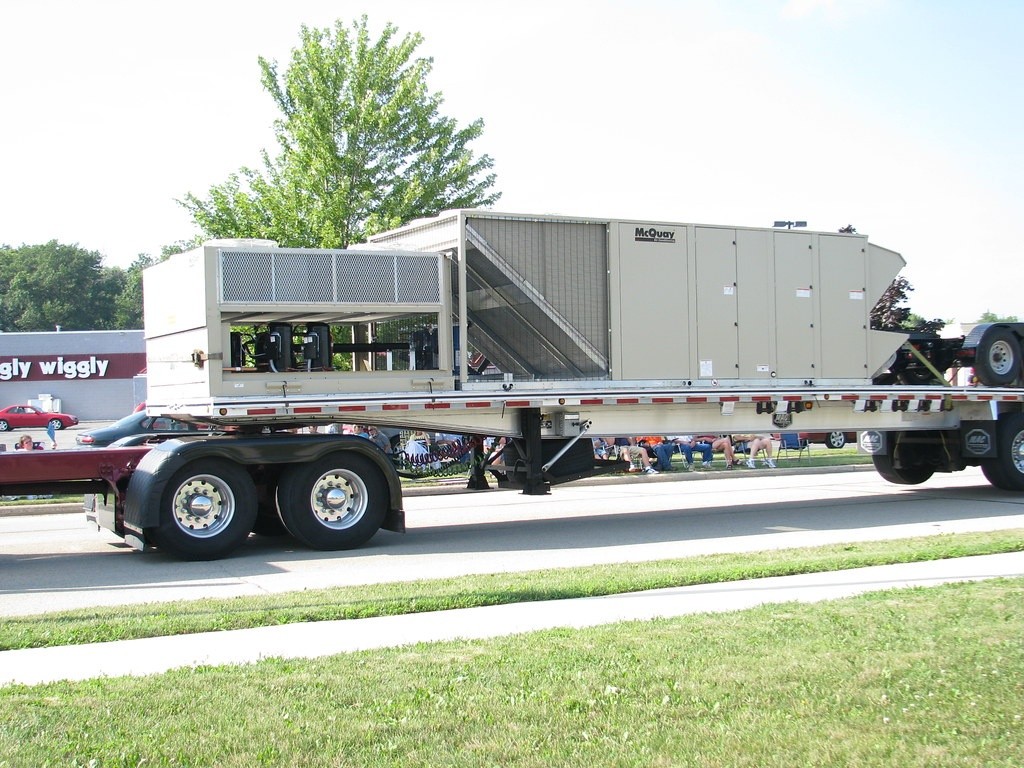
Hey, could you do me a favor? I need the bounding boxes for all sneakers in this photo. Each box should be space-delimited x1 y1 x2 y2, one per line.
766 458 776 467
745 459 756 468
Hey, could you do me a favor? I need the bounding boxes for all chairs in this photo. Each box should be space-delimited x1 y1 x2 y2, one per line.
776 433 811 465
590 432 772 470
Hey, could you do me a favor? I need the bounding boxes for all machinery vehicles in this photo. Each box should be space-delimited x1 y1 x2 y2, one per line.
0 209 1024 545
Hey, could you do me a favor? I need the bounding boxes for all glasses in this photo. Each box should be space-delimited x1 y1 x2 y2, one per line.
368 429 374 432
357 426 363 429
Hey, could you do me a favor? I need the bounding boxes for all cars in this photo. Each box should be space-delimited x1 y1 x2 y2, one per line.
75 412 224 447
772 431 857 450
0 404 79 433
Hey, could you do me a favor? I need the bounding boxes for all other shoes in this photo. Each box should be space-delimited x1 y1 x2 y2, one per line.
727 464 733 470
645 468 659 474
687 464 695 472
665 467 678 473
733 456 741 465
629 465 636 472
653 464 662 471
701 463 715 470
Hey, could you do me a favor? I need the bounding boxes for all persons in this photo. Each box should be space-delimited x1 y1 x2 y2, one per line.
18 435 37 451
592 434 776 474
288 423 507 478
968 368 977 386
